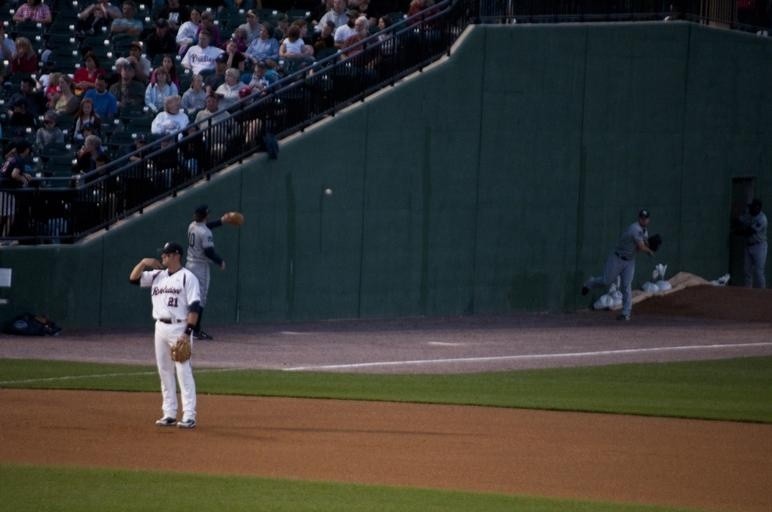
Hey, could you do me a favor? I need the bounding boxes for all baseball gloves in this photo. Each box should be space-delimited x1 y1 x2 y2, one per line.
170 334 191 361
647 234 661 251
224 212 244 226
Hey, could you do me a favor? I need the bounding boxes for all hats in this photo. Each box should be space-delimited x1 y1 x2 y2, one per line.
193 203 213 217
638 208 651 220
156 241 181 253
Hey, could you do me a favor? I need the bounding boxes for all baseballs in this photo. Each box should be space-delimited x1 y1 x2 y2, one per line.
325 189 332 195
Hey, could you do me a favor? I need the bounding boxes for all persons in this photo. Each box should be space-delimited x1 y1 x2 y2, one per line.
739 201 769 290
582 209 655 322
132 244 200 428
591 271 731 313
184 205 244 340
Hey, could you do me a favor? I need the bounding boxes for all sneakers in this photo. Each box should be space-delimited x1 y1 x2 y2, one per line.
615 314 632 322
177 418 196 428
155 416 176 426
194 331 213 341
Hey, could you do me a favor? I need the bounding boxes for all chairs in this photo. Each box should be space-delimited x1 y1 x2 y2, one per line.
1 1 407 188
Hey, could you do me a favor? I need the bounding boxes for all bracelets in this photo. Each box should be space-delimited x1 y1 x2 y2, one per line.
184 324 195 335
